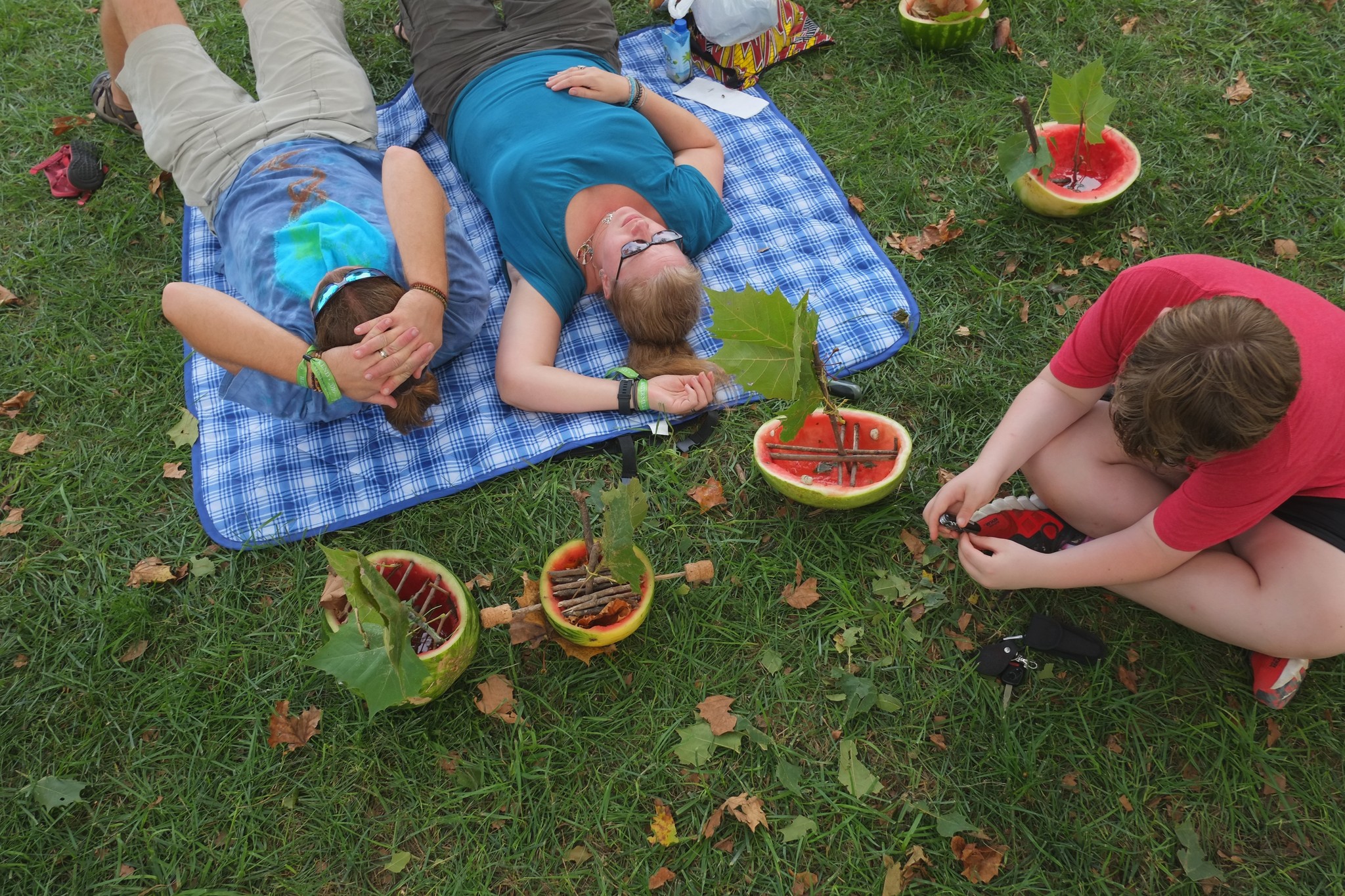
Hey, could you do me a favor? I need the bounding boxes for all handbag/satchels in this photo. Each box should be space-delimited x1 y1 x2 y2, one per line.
649 0 836 91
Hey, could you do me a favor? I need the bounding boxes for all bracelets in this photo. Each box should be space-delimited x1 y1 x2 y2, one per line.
620 75 647 111
310 350 324 394
617 378 636 416
632 380 640 411
604 366 650 411
296 345 342 404
410 283 449 311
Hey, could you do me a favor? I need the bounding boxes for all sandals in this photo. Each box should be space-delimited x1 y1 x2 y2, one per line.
391 18 413 48
91 70 144 137
28 139 110 205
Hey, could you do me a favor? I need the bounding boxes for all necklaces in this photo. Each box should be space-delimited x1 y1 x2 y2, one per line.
576 211 616 268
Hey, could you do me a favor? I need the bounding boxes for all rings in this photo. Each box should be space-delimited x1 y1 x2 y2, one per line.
578 65 586 71
691 408 696 413
378 348 389 359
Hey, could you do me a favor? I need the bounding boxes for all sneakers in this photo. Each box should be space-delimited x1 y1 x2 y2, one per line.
967 493 1093 555
1247 650 1312 710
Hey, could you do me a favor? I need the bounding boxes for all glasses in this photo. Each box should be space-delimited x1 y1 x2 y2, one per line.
614 229 683 290
312 267 406 336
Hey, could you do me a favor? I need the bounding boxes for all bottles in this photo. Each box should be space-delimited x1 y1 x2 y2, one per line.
661 19 693 85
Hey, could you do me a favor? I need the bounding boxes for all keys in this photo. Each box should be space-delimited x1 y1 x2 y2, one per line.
1001 662 1027 713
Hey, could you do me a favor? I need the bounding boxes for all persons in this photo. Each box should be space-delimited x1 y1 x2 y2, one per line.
399 0 733 416
87 0 451 436
922 253 1344 708
